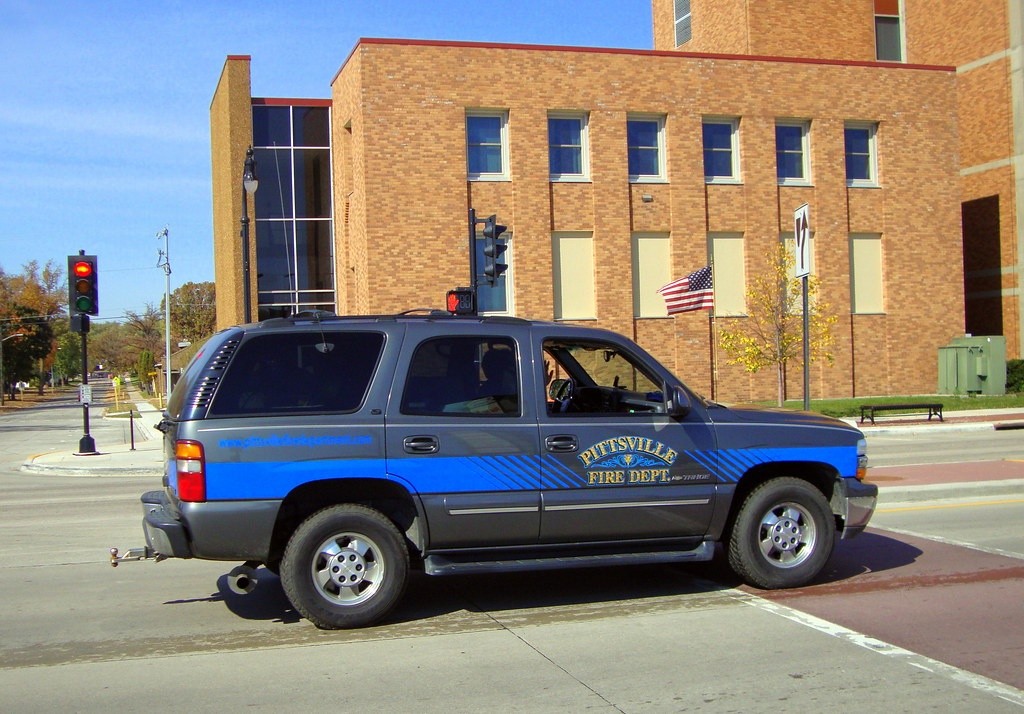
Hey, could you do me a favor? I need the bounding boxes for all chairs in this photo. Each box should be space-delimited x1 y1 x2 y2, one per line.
480 346 519 402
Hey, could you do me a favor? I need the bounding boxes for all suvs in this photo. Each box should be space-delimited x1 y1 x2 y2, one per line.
98 307 879 630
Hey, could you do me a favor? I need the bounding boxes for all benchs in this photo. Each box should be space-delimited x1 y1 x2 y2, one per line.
858 402 949 426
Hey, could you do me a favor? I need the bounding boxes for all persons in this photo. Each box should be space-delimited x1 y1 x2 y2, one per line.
544 360 553 386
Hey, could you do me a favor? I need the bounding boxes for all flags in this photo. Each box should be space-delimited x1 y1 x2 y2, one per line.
656 265 713 315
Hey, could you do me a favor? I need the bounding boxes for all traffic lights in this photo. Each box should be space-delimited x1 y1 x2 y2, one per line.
483 212 508 287
67 255 100 316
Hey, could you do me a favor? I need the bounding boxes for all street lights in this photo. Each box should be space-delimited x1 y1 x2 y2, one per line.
1 333 23 405
